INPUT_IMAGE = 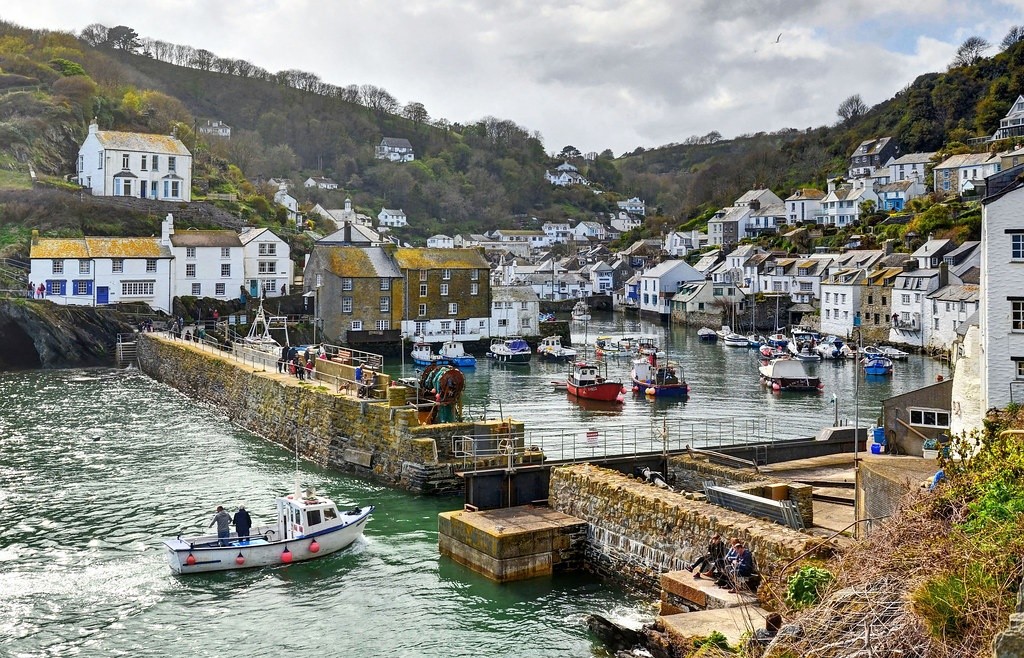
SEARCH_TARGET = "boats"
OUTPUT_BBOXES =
[439,330,478,368]
[565,360,623,401]
[245,290,306,359]
[758,351,821,393]
[716,326,731,339]
[548,380,568,391]
[630,352,688,398]
[489,335,532,366]
[787,323,910,360]
[571,302,592,321]
[698,325,718,343]
[410,336,449,368]
[161,432,375,576]
[862,356,895,375]
[596,336,665,359]
[537,334,578,362]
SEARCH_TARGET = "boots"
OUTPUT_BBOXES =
[703,570,713,577]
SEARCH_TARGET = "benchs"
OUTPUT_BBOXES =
[364,357,381,370]
[331,350,351,364]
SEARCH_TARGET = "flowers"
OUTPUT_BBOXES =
[922,437,942,451]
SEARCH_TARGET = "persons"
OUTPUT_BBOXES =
[262,288,267,299]
[807,337,816,356]
[232,505,251,543]
[277,342,326,380]
[185,330,192,341]
[281,284,287,296]
[797,340,803,353]
[685,534,754,594]
[167,316,184,338]
[199,327,206,345]
[138,317,155,332]
[355,363,379,398]
[29,281,45,299]
[209,506,232,547]
[213,309,219,329]
[193,325,201,343]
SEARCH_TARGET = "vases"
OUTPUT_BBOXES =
[923,449,939,460]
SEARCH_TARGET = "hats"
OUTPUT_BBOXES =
[238,503,245,509]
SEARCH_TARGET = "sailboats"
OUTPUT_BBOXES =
[396,335,421,388]
[725,281,791,349]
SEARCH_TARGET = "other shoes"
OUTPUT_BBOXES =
[714,579,727,586]
[693,572,700,578]
[685,567,694,573]
[728,588,736,593]
[722,585,729,589]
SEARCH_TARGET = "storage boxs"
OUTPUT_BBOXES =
[873,426,885,443]
[871,444,884,454]
